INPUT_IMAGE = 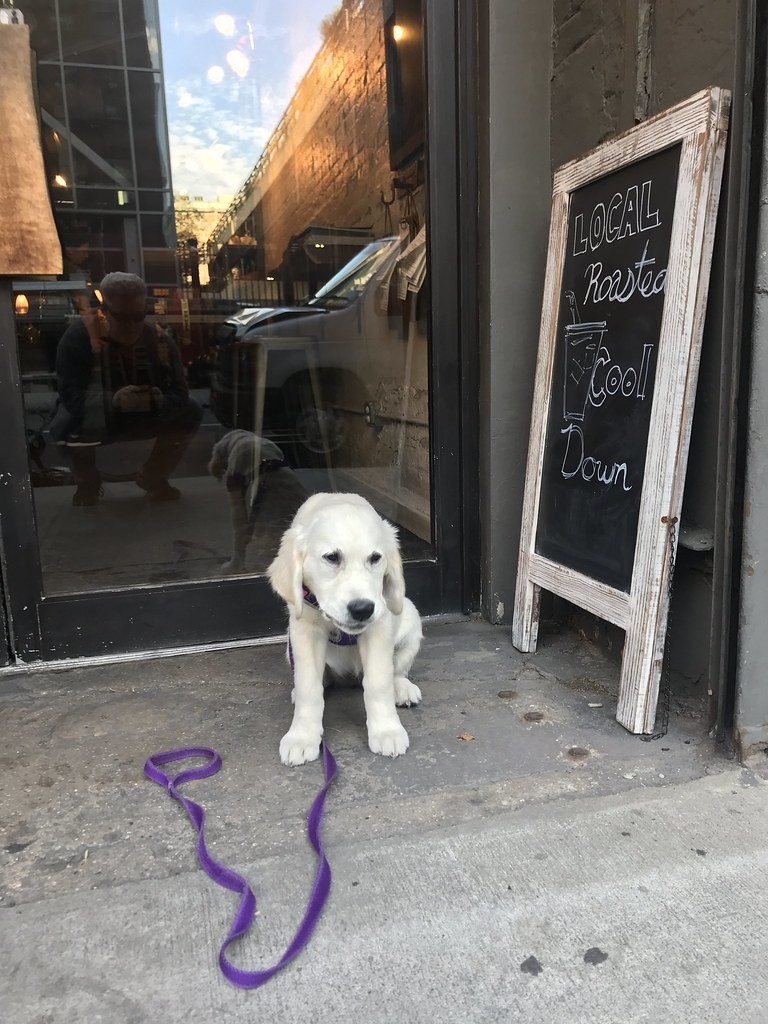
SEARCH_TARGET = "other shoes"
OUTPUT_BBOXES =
[135,464,182,500]
[72,474,105,507]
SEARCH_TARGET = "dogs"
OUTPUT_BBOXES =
[266,492,425,770]
[209,428,307,576]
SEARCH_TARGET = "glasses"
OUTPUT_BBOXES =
[103,302,149,323]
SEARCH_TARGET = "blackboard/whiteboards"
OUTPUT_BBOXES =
[508,82,735,633]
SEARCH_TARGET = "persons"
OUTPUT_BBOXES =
[51,272,204,505]
